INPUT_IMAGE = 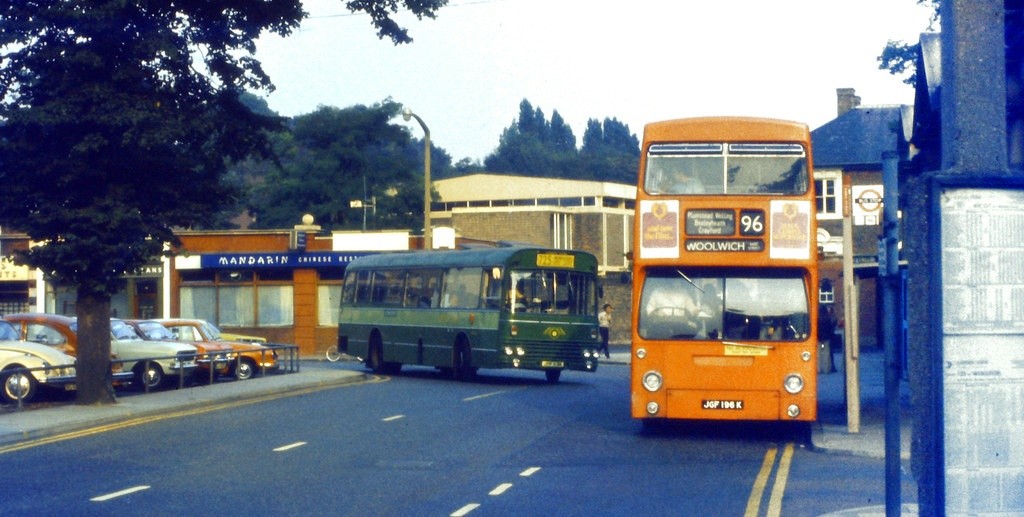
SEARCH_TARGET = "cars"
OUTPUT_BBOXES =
[69,317,199,389]
[125,318,279,380]
[0,319,78,403]
[3,314,124,390]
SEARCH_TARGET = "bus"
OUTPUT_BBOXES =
[336,239,603,381]
[626,116,831,444]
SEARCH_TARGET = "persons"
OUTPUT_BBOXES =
[597,304,613,358]
[492,280,524,306]
[701,284,721,312]
[817,305,837,374]
[667,164,705,194]
[718,282,761,340]
[646,278,698,319]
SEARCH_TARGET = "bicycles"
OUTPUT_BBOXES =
[326,336,363,363]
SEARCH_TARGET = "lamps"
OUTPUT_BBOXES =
[301,213,315,225]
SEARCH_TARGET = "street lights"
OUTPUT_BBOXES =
[402,107,431,250]
